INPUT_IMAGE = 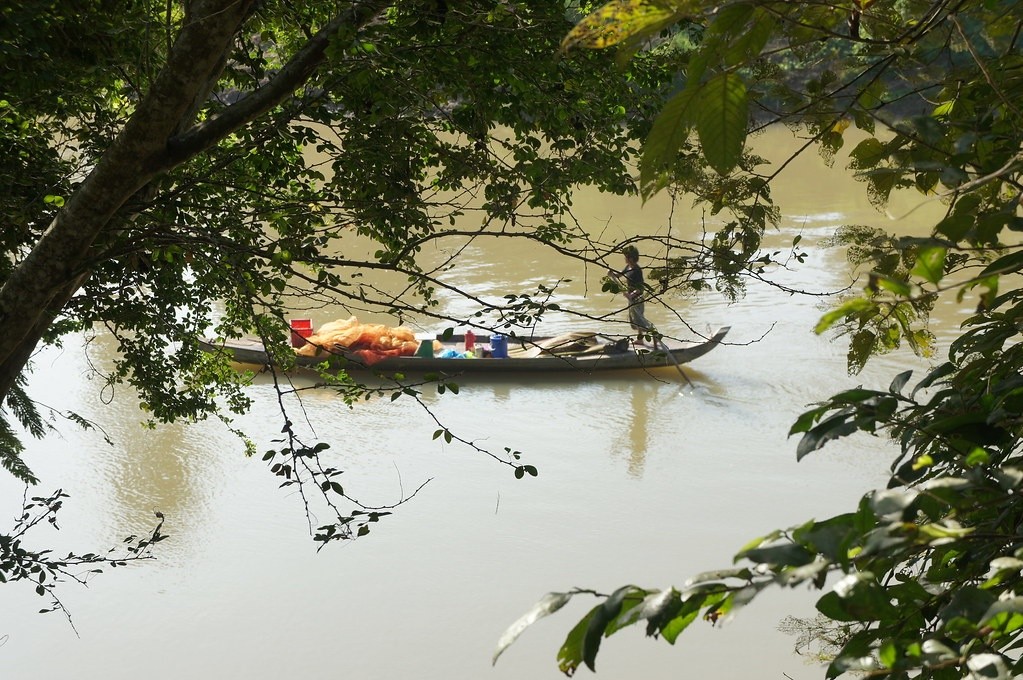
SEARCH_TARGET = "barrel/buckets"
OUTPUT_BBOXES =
[490,334,508,358]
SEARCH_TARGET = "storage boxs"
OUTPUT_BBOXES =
[289,318,313,348]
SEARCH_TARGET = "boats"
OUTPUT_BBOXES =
[195,325,731,374]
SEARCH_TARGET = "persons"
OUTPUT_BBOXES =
[607,247,658,349]
